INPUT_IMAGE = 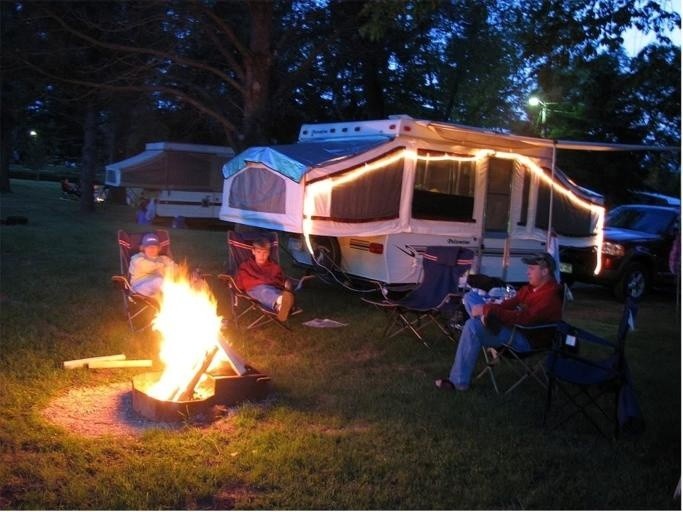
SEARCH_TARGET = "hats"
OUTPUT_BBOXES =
[521,252,555,270]
[142,233,160,245]
[254,237,272,248]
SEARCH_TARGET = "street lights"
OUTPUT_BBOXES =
[527,96,546,137]
[28,129,40,182]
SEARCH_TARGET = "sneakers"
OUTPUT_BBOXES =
[276,291,303,322]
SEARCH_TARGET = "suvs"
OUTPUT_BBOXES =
[559,204,681,302]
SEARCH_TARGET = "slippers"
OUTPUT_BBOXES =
[434,379,454,390]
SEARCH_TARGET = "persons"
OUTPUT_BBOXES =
[127,233,181,299]
[62,178,81,199]
[235,236,296,322]
[432,251,563,392]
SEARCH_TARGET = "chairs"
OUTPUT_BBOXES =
[378,246,474,351]
[472,284,567,398]
[111,230,173,333]
[544,295,642,443]
[218,229,316,335]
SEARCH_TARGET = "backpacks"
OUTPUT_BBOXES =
[1,216,28,225]
